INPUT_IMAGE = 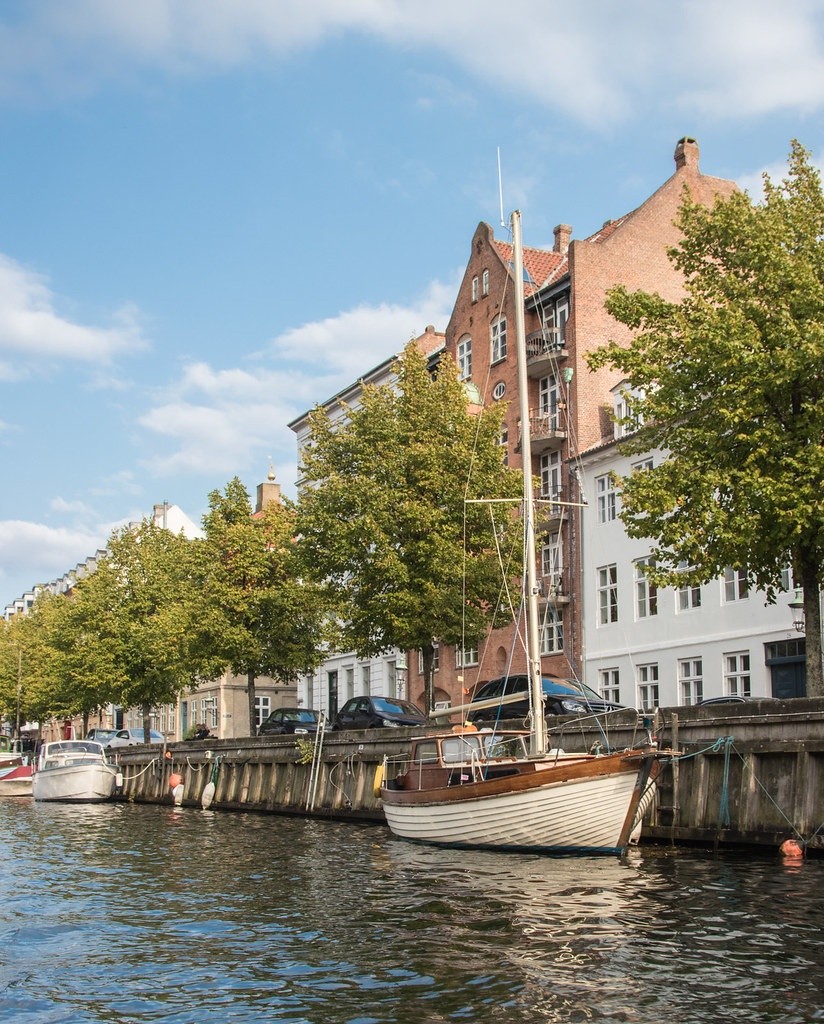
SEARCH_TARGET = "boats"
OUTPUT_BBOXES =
[1,754,33,796]
[31,739,119,802]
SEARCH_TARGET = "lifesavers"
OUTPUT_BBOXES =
[373,764,384,798]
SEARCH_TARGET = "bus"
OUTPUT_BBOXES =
[0,734,11,754]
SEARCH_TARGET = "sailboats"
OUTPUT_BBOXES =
[374,200,688,852]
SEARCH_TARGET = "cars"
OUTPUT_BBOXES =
[106,727,173,748]
[84,728,121,747]
[256,708,332,736]
[694,696,781,706]
[332,696,431,732]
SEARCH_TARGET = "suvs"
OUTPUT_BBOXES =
[465,673,629,723]
